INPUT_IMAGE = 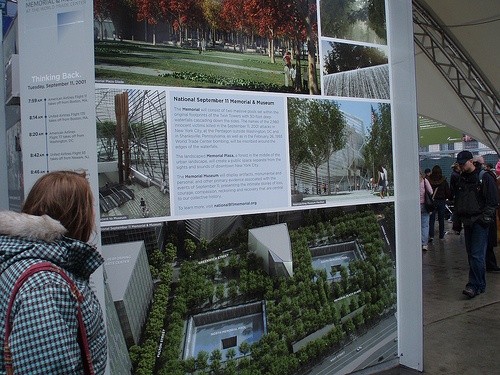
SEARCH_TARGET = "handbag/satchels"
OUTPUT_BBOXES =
[425,190,435,212]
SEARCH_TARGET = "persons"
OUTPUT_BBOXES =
[289,64,296,89]
[419,167,433,250]
[114,139,172,217]
[376,166,385,199]
[452,150,499,298]
[423,168,431,181]
[0,171,108,375]
[94,19,282,59]
[428,165,452,242]
[301,67,309,91]
[293,165,373,199]
[283,51,291,87]
[473,154,500,271]
[449,163,462,208]
[381,166,389,198]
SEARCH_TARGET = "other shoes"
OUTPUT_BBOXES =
[439,237,446,240]
[422,245,428,250]
[455,231,460,235]
[462,282,486,297]
[429,238,433,242]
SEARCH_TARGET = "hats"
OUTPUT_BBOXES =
[456,150,473,164]
